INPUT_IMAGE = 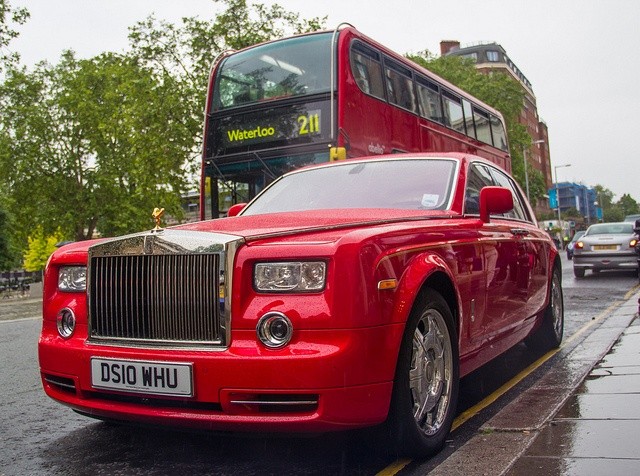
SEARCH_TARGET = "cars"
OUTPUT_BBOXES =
[573,221,640,278]
[37,151,565,459]
[566,231,586,260]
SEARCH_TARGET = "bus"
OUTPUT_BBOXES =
[198,22,513,221]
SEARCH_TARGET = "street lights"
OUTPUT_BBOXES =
[553,163,573,225]
[521,139,545,202]
